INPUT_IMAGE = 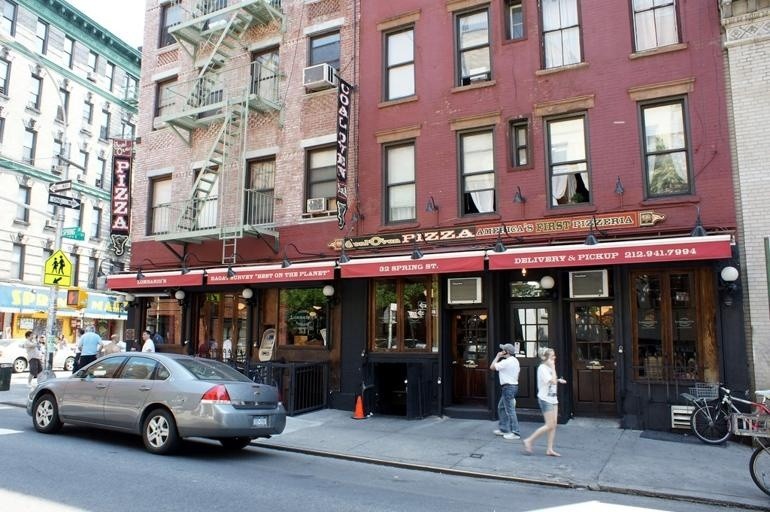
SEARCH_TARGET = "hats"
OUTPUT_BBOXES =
[499,343,514,354]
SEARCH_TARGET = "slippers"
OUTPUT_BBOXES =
[546,452,561,457]
[522,439,535,455]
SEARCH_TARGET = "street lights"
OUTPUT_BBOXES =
[1,29,72,385]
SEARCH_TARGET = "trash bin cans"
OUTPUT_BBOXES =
[0,363,12,390]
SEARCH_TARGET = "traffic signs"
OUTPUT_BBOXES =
[417,310,425,317]
[47,179,73,194]
[65,232,85,240]
[418,302,427,309]
[60,227,82,236]
[47,195,82,211]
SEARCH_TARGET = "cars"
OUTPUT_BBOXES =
[0,333,75,373]
[375,338,427,350]
[20,350,289,456]
[70,338,126,371]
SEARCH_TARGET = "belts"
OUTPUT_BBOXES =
[501,384,517,387]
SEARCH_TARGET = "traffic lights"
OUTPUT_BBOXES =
[66,286,89,310]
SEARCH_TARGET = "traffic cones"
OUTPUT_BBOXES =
[349,393,367,420]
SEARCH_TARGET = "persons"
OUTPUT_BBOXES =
[523,347,567,456]
[21,322,163,379]
[200,335,233,361]
[490,344,522,440]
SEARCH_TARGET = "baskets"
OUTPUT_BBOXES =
[680,382,720,401]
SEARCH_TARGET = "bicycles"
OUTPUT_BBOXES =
[680,377,770,445]
[742,427,770,497]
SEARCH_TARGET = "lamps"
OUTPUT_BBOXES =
[322,284,337,307]
[118,293,138,311]
[494,222,523,253]
[425,174,624,215]
[175,290,189,307]
[687,204,707,238]
[96,237,382,282]
[583,216,608,246]
[411,231,429,259]
[719,262,743,307]
[540,275,555,296]
[242,289,255,305]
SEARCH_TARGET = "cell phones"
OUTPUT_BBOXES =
[503,351,507,355]
[547,359,550,362]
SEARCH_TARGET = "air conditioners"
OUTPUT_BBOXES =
[302,62,335,90]
[307,198,328,212]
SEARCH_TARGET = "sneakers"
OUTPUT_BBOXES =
[493,429,508,435]
[503,433,520,440]
[27,384,32,388]
